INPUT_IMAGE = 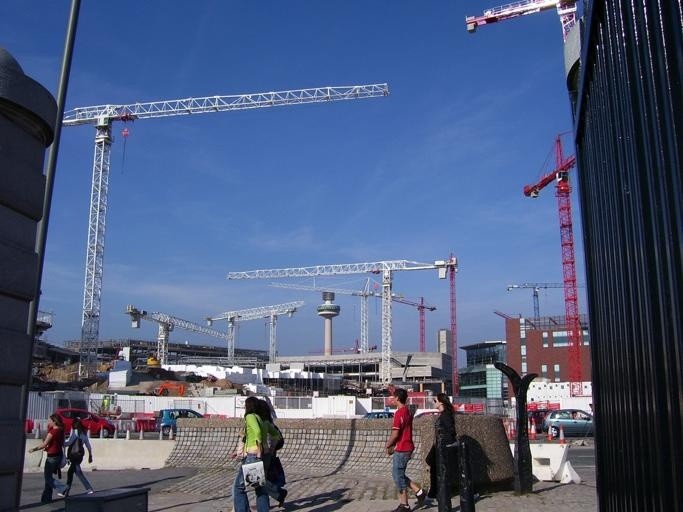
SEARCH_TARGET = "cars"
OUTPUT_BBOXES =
[530,409,594,437]
[155,409,206,436]
[362,412,394,419]
[47,407,115,438]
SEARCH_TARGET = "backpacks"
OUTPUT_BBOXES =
[252,412,285,458]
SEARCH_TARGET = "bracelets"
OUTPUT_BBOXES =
[32,447,40,451]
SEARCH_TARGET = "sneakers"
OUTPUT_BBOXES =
[391,503,411,512]
[415,487,427,507]
[57,485,95,497]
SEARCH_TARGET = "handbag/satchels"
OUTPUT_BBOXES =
[241,460,267,492]
[66,435,85,463]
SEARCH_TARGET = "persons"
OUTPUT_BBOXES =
[239,400,288,510]
[385,387,427,512]
[232,396,269,512]
[431,393,476,512]
[57,419,94,498]
[28,414,69,504]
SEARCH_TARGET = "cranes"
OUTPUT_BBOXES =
[124,305,227,367]
[225,258,437,392]
[464,0,582,41]
[268,277,377,354]
[493,130,583,404]
[205,301,306,363]
[434,251,461,397]
[61,79,392,391]
[393,294,437,352]
